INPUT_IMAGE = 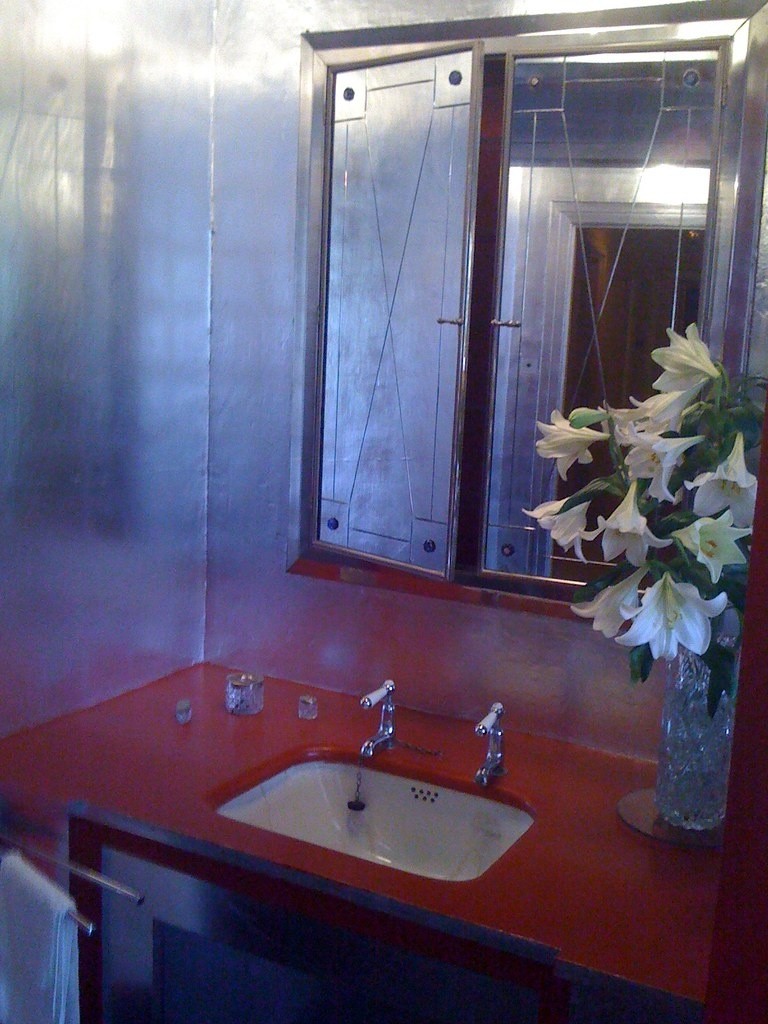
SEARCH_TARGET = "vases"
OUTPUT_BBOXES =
[654,599,744,829]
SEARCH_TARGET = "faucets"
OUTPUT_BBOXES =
[359,677,396,756]
[473,701,509,786]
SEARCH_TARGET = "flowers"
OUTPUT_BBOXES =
[523,316,767,715]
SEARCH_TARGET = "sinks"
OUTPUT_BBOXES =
[216,743,535,882]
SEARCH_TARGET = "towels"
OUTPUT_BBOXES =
[1,854,80,1024]
